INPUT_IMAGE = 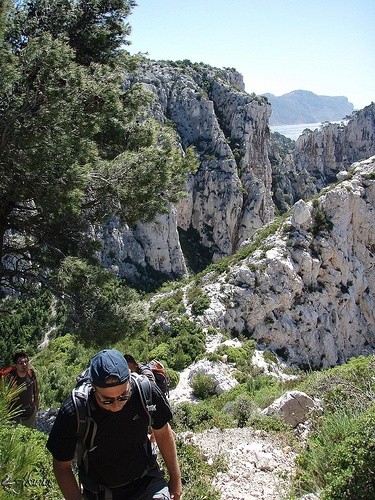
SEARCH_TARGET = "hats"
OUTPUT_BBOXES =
[88,350,130,388]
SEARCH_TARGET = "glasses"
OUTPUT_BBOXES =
[17,360,28,365]
[95,389,130,405]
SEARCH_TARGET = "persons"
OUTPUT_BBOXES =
[123,354,157,450]
[46,349,182,500]
[4,352,40,428]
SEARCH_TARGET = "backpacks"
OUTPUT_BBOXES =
[72,367,161,497]
[138,359,167,393]
[0,365,34,394]
